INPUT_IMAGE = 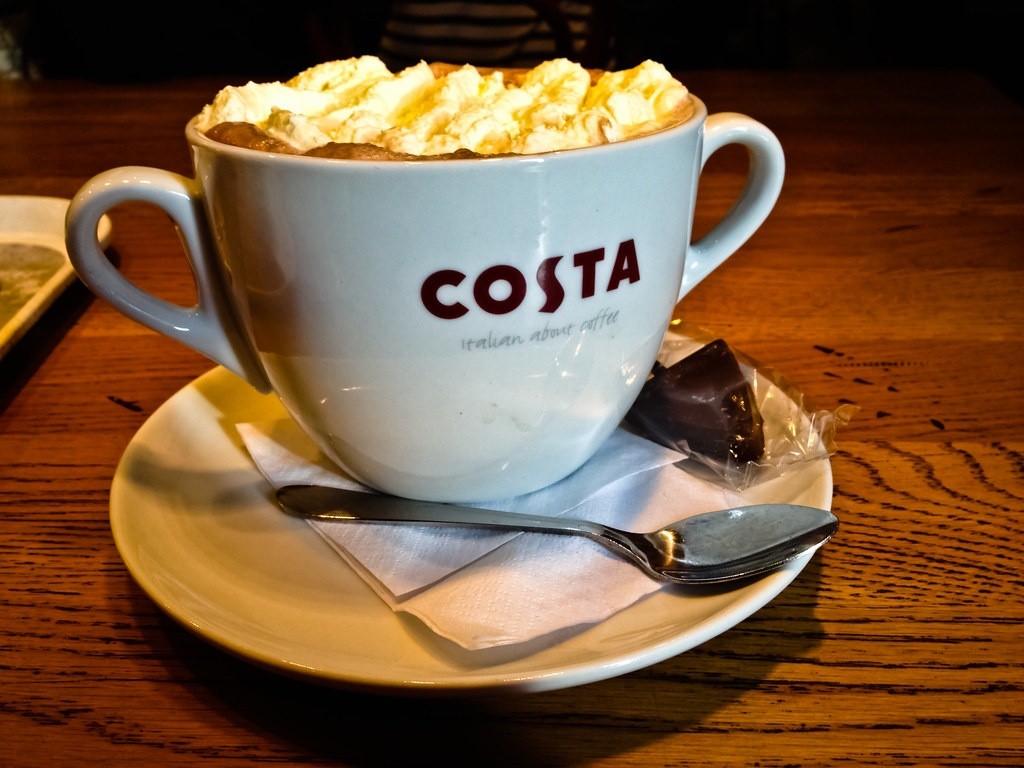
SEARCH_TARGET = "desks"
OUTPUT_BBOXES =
[1,76,1024,768]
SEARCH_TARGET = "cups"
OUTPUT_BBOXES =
[65,91,786,503]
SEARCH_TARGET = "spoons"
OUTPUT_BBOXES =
[275,483,842,587]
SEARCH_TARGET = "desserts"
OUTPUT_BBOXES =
[619,338,767,465]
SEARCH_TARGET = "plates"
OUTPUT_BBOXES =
[0,195,112,355]
[107,330,835,699]
